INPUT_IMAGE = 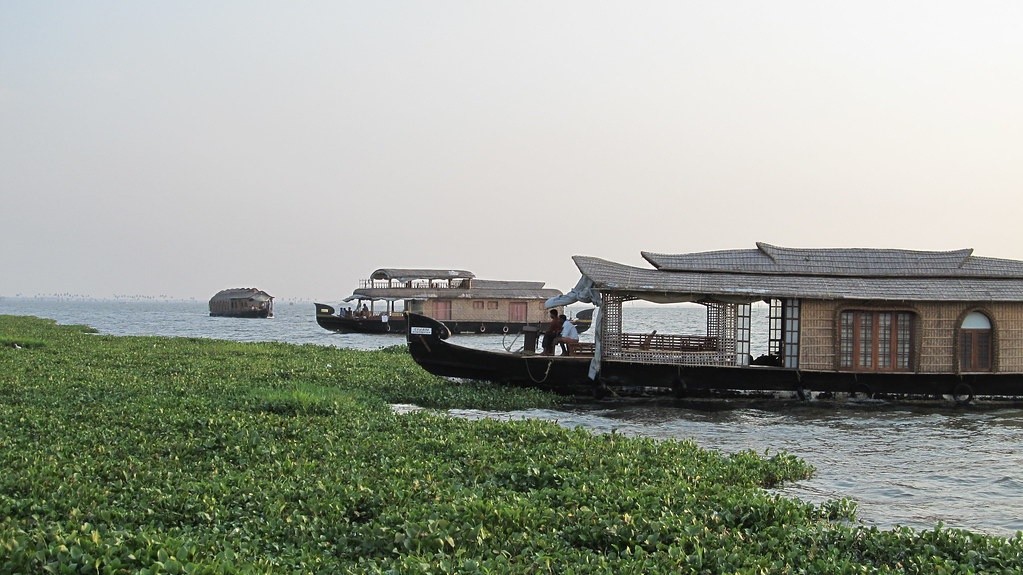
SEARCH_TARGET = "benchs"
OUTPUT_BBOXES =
[567,329,718,357]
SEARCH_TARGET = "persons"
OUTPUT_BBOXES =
[544,313,579,356]
[540,308,563,356]
[340,303,370,319]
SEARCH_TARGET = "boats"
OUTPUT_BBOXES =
[314,268,597,338]
[208,287,274,318]
[400,239,1023,408]
